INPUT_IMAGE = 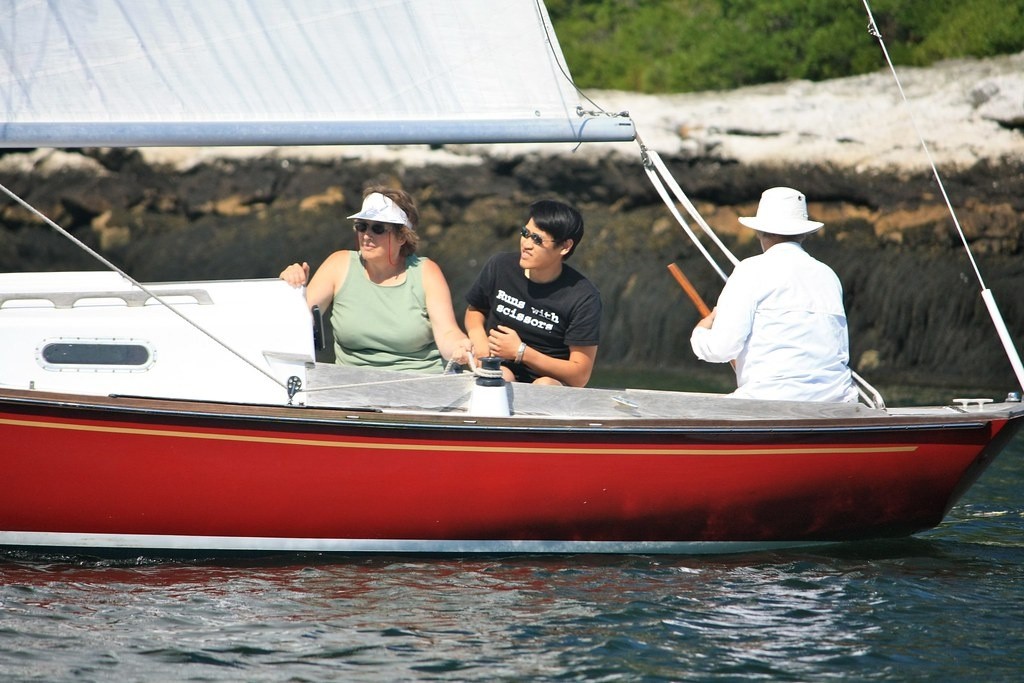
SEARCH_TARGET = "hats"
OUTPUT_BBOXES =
[738,186,824,235]
[347,192,413,231]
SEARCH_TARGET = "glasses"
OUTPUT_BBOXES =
[520,226,564,246]
[354,222,402,235]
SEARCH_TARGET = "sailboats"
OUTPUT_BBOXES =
[0,1,1023,558]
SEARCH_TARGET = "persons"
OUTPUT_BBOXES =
[464,200,602,388]
[690,186,858,402]
[279,186,475,375]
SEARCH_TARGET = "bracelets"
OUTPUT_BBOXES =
[514,342,526,364]
